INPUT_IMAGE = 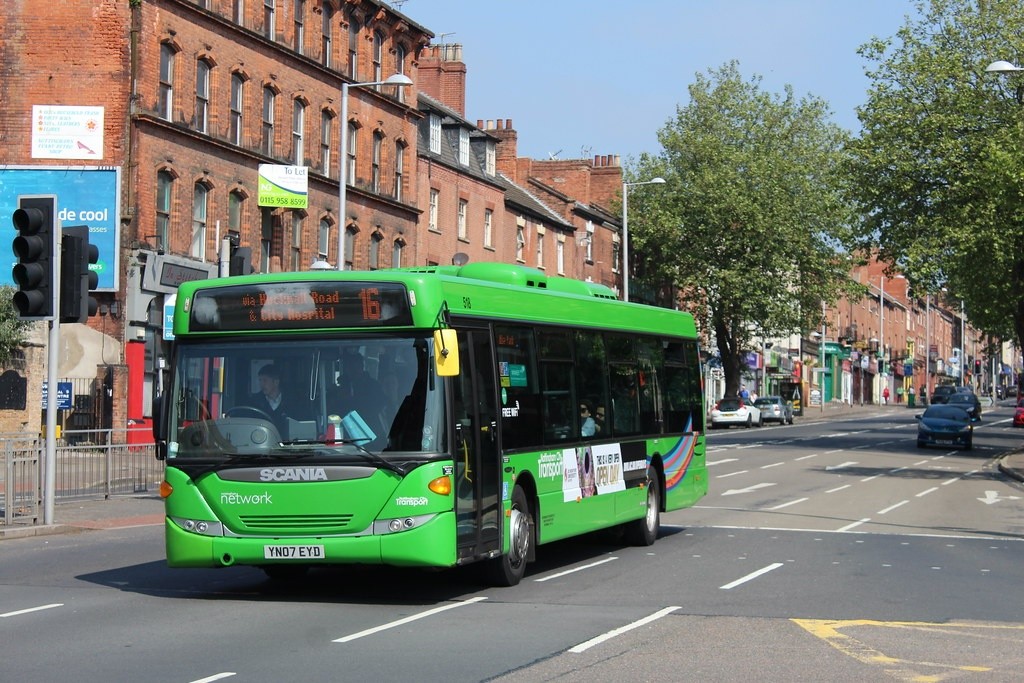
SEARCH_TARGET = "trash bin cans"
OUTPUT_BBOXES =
[908,394,914,406]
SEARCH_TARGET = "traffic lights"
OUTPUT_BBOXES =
[974,359,981,376]
[61,223,98,322]
[12,194,53,321]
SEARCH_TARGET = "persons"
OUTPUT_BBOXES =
[738,384,757,403]
[223,363,309,443]
[897,384,926,403]
[332,352,647,448]
[883,386,889,405]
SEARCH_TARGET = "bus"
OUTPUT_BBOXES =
[157,260,708,587]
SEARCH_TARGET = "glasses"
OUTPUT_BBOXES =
[581,408,588,413]
[595,412,605,418]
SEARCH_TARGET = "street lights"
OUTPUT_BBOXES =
[619,175,668,302]
[339,73,415,267]
[878,273,908,406]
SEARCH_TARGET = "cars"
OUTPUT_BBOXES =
[752,396,794,426]
[712,397,763,429]
[929,385,981,421]
[914,403,974,449]
